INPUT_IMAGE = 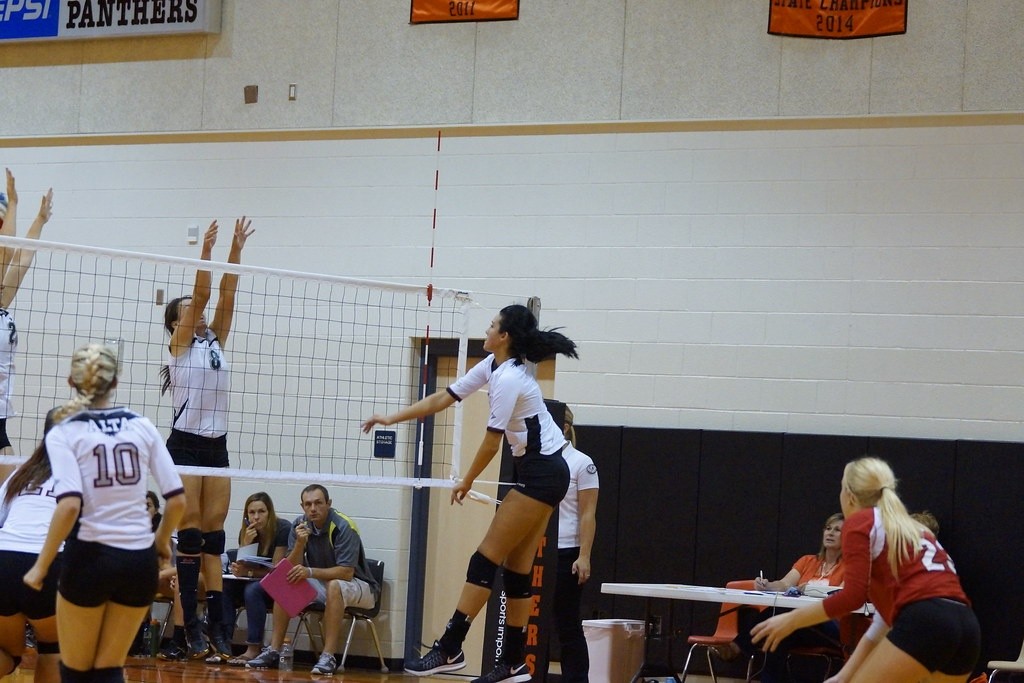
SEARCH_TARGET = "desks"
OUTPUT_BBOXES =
[600,582,876,683]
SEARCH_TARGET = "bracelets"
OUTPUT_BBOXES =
[307,567,312,578]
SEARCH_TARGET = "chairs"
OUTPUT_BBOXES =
[133,536,389,675]
[680,577,1024,683]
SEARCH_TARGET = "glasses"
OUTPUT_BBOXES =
[210,350,220,370]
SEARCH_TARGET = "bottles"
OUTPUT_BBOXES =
[279,638,293,672]
[146,619,160,656]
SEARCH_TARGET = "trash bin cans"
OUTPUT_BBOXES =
[581,619,645,683]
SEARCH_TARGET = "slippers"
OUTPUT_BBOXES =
[204,655,224,664]
[228,655,249,667]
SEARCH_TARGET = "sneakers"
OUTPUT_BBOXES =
[310,652,336,675]
[184,617,208,658]
[157,647,186,660]
[470,656,532,683]
[208,622,231,659]
[404,640,467,676]
[245,646,279,669]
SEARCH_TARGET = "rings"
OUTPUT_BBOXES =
[587,575,590,577]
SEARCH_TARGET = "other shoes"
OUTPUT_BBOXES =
[710,642,739,664]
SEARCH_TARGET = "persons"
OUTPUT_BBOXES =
[159,216,256,658]
[362,304,578,683]
[707,513,846,663]
[543,404,599,683]
[750,456,982,683]
[0,404,64,683]
[0,165,53,487]
[246,485,381,677]
[910,511,939,535]
[126,491,207,663]
[23,343,186,683]
[206,492,292,666]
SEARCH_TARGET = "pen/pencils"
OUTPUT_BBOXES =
[743,592,763,596]
[760,570,762,581]
[244,518,249,526]
